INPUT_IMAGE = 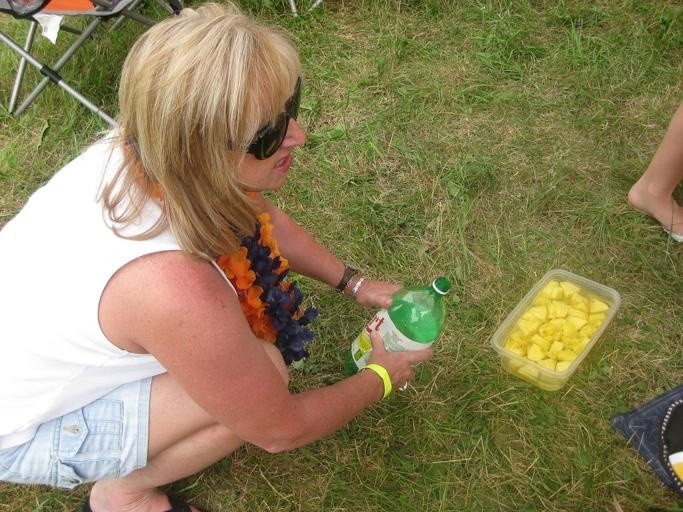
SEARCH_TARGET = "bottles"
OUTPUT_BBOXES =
[343,277,451,376]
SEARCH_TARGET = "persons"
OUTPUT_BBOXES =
[626,97,682,245]
[0,0,438,511]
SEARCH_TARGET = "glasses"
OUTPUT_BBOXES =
[227,74,302,161]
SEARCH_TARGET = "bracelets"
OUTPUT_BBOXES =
[347,275,369,300]
[333,257,361,291]
[360,362,394,402]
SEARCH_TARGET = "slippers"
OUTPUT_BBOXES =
[83,493,211,512]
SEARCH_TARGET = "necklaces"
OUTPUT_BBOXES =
[121,126,320,367]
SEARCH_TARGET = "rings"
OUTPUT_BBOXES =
[397,381,408,391]
